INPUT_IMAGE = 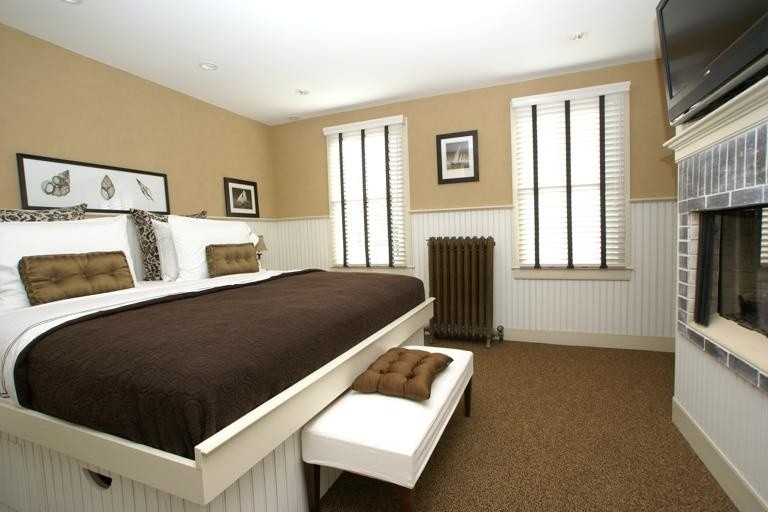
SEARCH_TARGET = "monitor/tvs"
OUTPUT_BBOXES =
[655,1,768,126]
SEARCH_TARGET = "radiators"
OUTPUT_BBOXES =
[424,236,504,348]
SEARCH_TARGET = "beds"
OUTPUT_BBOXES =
[0,269,437,512]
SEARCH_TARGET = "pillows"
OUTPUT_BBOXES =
[0,203,260,308]
[351,346,454,400]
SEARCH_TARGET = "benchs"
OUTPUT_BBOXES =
[299,344,473,512]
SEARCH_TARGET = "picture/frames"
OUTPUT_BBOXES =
[223,177,259,218]
[16,153,170,215]
[436,130,479,184]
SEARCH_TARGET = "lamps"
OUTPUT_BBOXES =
[255,236,268,260]
